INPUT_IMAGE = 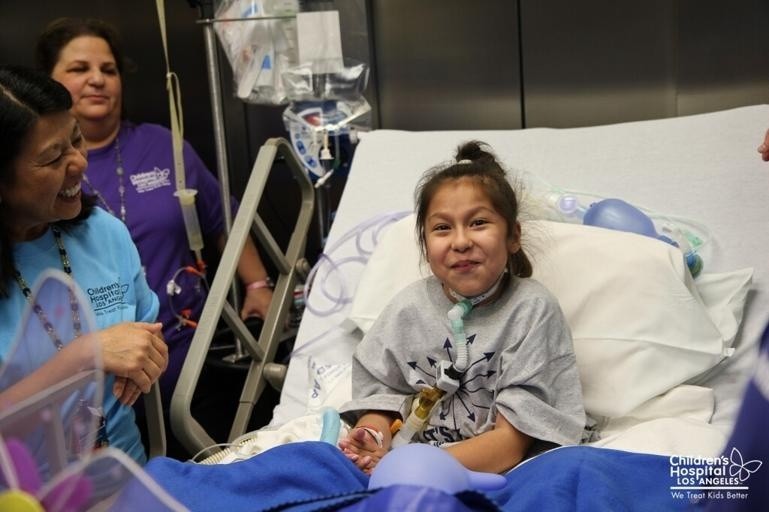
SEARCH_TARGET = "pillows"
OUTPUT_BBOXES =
[353,211,735,420]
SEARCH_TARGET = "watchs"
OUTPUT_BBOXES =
[247,276,275,290]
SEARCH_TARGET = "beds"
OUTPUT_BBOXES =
[0,104,769,511]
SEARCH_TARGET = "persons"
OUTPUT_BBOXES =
[339,140,586,474]
[34,16,273,393]
[0,65,169,512]
